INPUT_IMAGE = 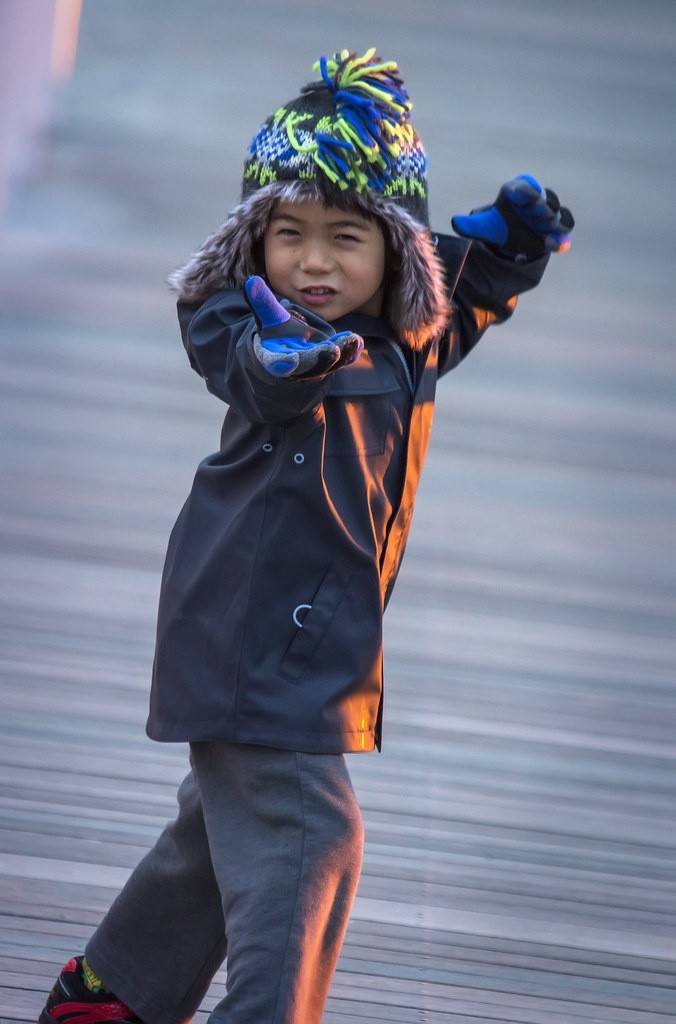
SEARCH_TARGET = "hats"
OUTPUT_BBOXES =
[167,46,451,353]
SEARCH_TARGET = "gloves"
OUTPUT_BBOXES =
[450,174,575,262]
[245,277,364,377]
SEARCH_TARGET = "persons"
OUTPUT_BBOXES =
[36,46,577,1024]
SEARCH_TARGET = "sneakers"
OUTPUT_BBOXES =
[40,957,142,1024]
